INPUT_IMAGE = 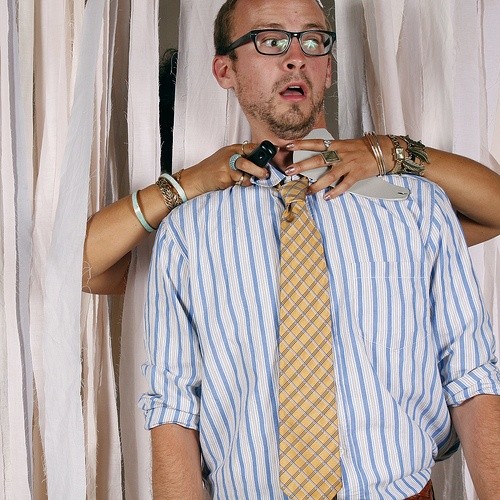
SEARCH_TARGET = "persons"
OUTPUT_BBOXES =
[82,48,500,297]
[144,0,500,500]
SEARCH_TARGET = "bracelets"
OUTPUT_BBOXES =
[398,135,431,178]
[132,188,158,234]
[155,167,188,211]
[363,131,386,177]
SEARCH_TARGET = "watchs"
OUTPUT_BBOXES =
[386,134,405,175]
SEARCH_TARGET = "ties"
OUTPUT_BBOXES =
[277,176,342,500]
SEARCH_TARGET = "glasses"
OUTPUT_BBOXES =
[228,28,336,57]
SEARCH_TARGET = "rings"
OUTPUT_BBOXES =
[235,173,245,185]
[320,150,342,164]
[229,153,243,168]
[322,138,333,150]
[241,138,248,153]
